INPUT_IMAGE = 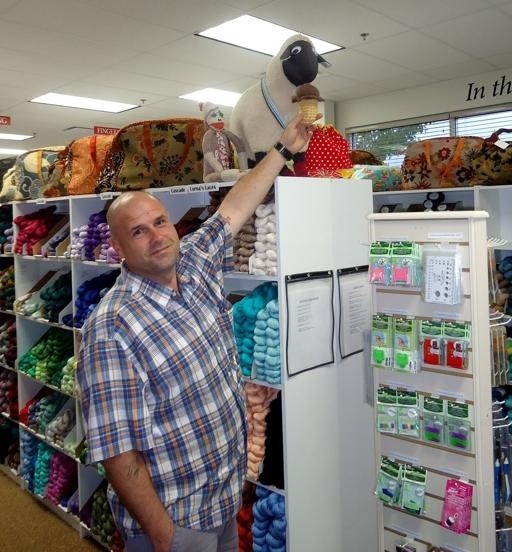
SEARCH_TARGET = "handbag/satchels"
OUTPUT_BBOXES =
[95,115,216,193]
[400,135,489,190]
[0,132,114,203]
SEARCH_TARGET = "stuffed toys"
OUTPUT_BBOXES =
[199,108,253,185]
[225,33,333,172]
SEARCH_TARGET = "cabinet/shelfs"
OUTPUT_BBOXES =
[0,174,371,551]
[371,187,512,551]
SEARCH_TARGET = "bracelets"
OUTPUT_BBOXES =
[273,142,293,161]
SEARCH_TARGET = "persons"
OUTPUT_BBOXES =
[70,110,328,552]
